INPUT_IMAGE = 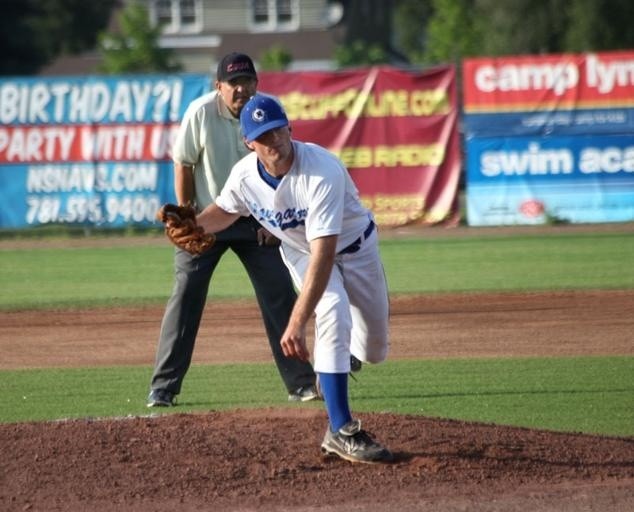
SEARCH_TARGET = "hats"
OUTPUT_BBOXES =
[215,51,257,83]
[237,94,289,143]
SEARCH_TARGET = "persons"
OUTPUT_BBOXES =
[165,97,392,465]
[146,51,324,407]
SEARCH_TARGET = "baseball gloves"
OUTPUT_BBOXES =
[158,203,218,256]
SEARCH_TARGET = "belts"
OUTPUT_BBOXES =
[335,220,375,255]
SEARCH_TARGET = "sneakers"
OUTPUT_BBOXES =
[146,388,176,407]
[349,353,361,371]
[286,383,318,402]
[319,421,394,465]
